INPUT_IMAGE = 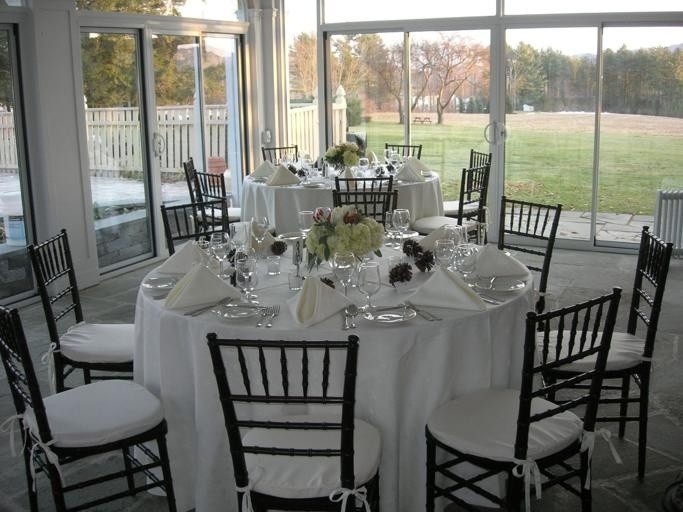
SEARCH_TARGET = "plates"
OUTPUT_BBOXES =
[386,231,419,239]
[362,309,416,324]
[252,177,266,182]
[421,171,431,177]
[475,277,526,291]
[279,231,302,241]
[215,303,258,320]
[142,278,178,290]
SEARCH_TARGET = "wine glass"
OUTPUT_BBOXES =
[332,251,355,297]
[196,222,259,305]
[250,217,268,260]
[386,209,410,250]
[357,262,381,310]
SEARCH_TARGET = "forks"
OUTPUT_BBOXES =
[405,300,443,321]
[255,304,280,327]
[471,286,505,302]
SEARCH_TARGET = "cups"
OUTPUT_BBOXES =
[435,224,478,288]
[287,272,302,295]
[266,255,281,275]
[298,211,313,240]
[271,148,403,188]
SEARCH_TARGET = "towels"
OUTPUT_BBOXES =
[401,268,488,316]
[286,275,354,329]
[162,264,248,311]
[156,238,223,273]
[475,247,534,279]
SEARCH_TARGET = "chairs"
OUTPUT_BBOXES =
[24,227,147,393]
[496,194,564,333]
[530,224,675,485]
[425,285,622,512]
[204,332,384,511]
[0,305,177,511]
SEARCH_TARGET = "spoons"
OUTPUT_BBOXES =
[183,296,231,316]
[348,303,358,328]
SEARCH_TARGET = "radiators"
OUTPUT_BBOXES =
[652,190,683,258]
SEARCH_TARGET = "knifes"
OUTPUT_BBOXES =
[342,305,350,329]
